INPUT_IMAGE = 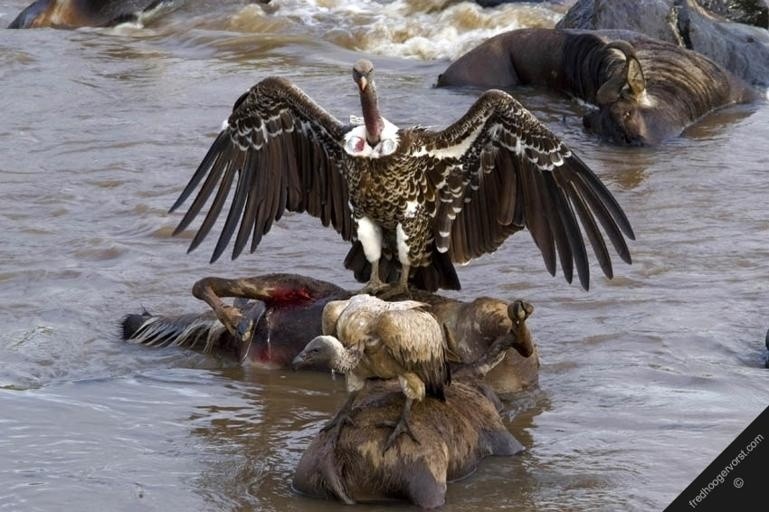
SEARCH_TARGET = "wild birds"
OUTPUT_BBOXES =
[167,59,636,299]
[293,294,451,456]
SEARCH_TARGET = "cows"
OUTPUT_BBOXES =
[293,347,540,508]
[122,272,540,395]
[434,29,769,147]
